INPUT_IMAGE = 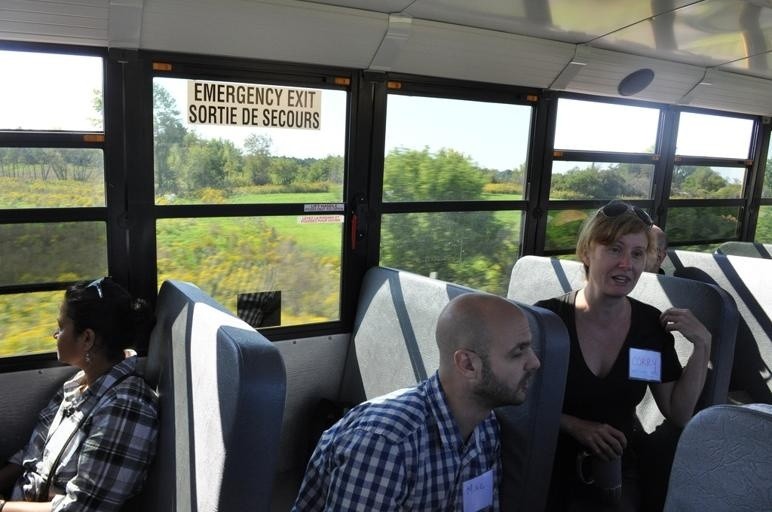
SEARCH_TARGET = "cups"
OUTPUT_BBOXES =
[575,449,623,496]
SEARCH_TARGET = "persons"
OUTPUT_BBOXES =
[0,274,161,512]
[530,198,714,511]
[645,223,669,277]
[289,291,543,512]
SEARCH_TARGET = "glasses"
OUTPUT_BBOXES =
[603,202,655,228]
[86,276,113,299]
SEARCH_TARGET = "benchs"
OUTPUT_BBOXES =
[505,255,739,416]
[720,240,772,259]
[665,404,771,511]
[660,250,772,403]
[144,281,286,512]
[335,267,571,512]
